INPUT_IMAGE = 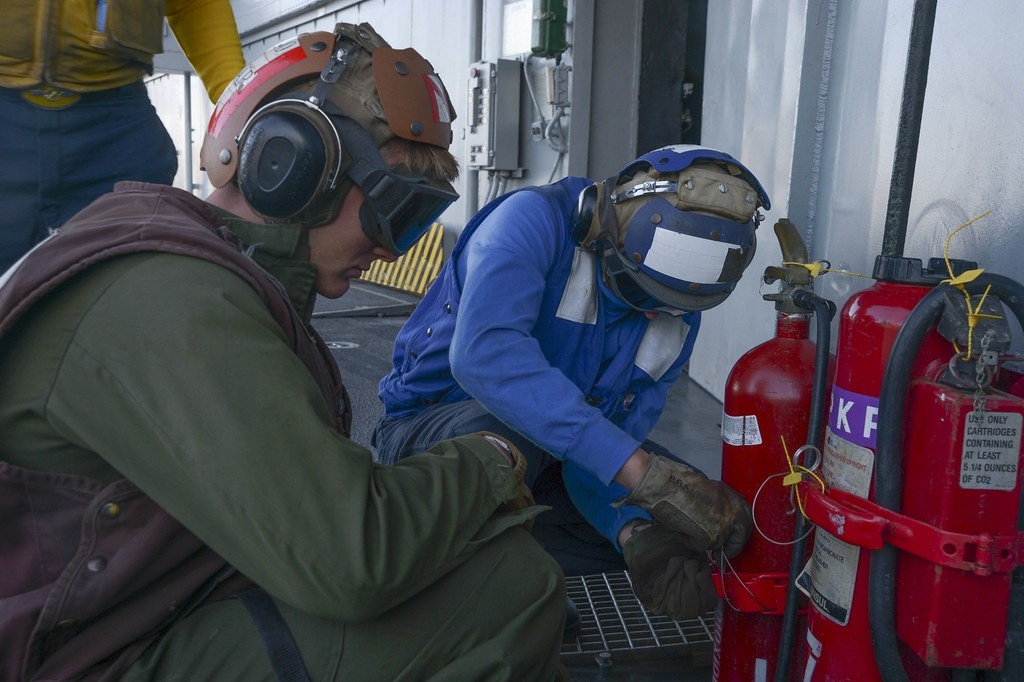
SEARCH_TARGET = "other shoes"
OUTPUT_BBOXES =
[563,594,582,640]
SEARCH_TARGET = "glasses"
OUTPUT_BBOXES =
[607,270,737,316]
[362,168,460,256]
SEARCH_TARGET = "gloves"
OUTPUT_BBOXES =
[622,519,720,621]
[475,431,536,532]
[611,451,752,564]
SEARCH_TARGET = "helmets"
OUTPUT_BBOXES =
[569,143,771,317]
[201,23,457,228]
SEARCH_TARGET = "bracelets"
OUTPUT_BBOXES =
[483,435,516,468]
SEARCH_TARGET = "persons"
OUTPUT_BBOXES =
[0,0,244,279]
[375,144,770,641]
[0,23,567,682]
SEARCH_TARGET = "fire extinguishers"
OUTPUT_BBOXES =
[717,218,1024,682]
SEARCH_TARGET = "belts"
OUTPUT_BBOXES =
[0,81,148,110]
